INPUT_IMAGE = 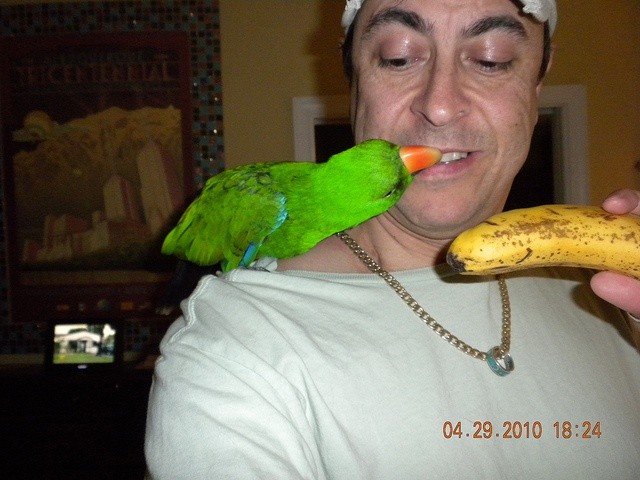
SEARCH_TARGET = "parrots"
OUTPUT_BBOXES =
[160,139,443,274]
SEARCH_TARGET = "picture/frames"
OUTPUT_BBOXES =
[0,30,196,324]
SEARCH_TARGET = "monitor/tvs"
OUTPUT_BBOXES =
[44,317,123,370]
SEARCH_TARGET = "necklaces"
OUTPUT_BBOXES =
[335,230,514,376]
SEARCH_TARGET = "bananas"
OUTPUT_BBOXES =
[445,205,640,279]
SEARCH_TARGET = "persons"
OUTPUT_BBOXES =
[142,0,640,480]
